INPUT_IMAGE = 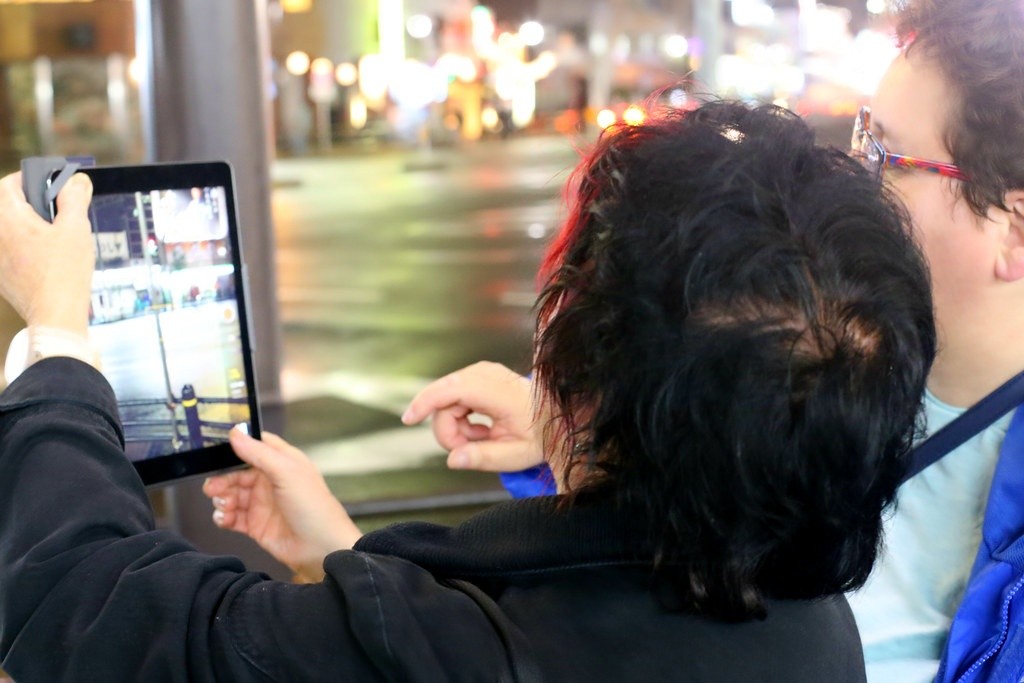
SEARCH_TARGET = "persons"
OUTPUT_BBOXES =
[0,100,935,683]
[185,185,215,236]
[400,0,1024,683]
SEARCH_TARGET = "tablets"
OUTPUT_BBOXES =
[45,159,263,488]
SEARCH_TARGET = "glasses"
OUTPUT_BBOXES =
[851,106,977,191]
